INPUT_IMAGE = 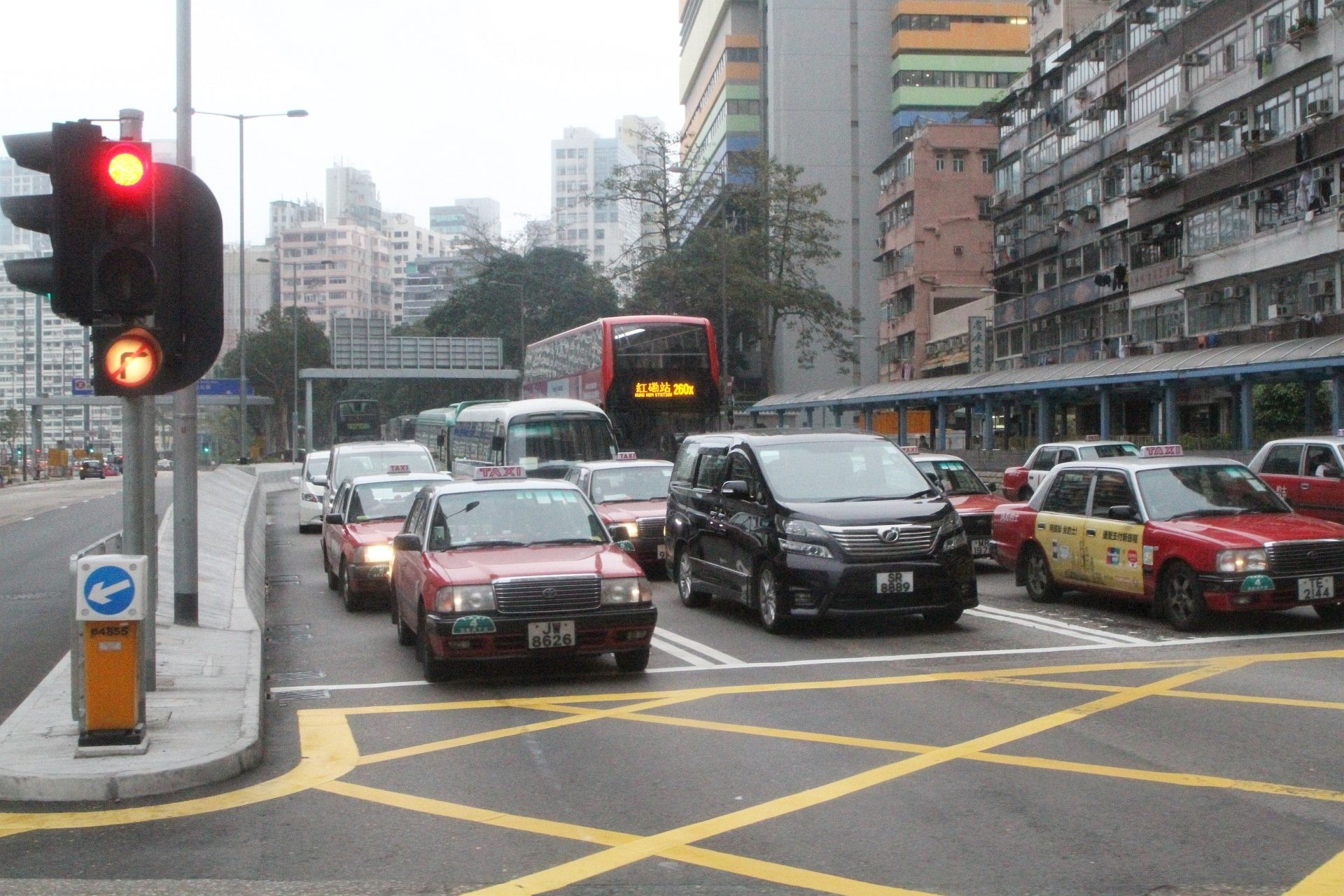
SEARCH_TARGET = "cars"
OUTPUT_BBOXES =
[664,427,980,639]
[290,449,379,534]
[1002,439,1144,499]
[393,465,659,681]
[893,444,1011,560]
[79,461,105,480]
[989,440,1344,630]
[1244,436,1344,519]
[323,471,460,613]
[564,451,677,578]
[323,440,440,518]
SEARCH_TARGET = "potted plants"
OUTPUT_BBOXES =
[1288,14,1316,39]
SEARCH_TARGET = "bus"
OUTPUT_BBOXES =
[523,315,725,461]
[106,452,122,472]
[330,397,379,446]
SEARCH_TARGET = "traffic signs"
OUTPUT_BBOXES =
[74,555,148,620]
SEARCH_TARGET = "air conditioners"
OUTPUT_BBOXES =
[1177,257,1190,270]
[1189,124,1209,141]
[1312,166,1333,182]
[1224,286,1237,299]
[904,335,969,356]
[954,246,963,254]
[988,9,1147,371]
[1330,193,1344,206]
[1198,292,1214,304]
[1308,279,1332,297]
[1324,0,1343,9]
[1151,223,1165,238]
[876,237,901,257]
[1248,189,1270,202]
[1158,91,1189,127]
[1229,110,1242,124]
[1233,195,1248,208]
[1240,128,1264,145]
[1177,53,1199,66]
[1307,99,1333,118]
[1163,140,1180,154]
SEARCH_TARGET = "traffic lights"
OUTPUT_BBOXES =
[18,448,21,456]
[89,142,160,314]
[36,448,40,453]
[1,118,87,322]
[176,101,309,462]
[203,442,208,453]
[88,444,94,453]
[92,324,166,395]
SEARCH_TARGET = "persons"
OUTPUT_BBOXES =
[919,435,929,448]
[1315,448,1339,477]
[358,486,386,516]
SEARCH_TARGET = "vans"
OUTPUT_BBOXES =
[381,407,452,470]
[452,396,620,482]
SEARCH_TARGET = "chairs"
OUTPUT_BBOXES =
[685,346,702,368]
[622,346,638,369]
[350,487,384,522]
[339,458,365,485]
[311,463,325,475]
[662,349,683,369]
[815,455,854,497]
[1267,457,1292,474]
[1307,453,1330,479]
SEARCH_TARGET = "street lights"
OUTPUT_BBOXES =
[257,257,335,460]
